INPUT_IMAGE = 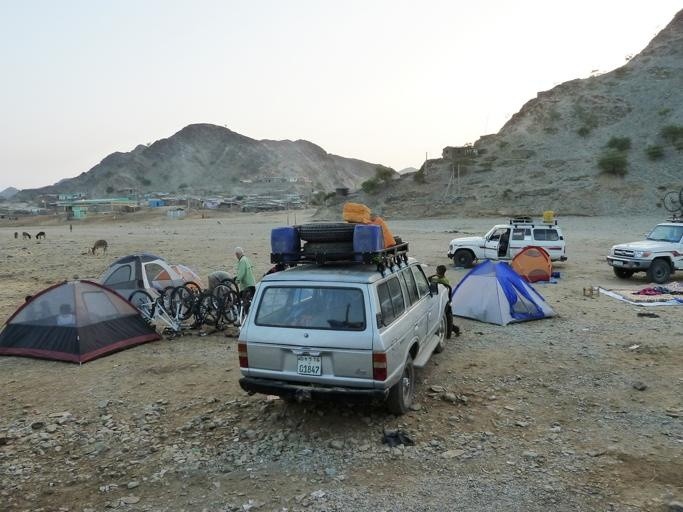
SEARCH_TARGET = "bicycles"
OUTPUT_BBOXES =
[658,187,682,217]
[124,277,259,341]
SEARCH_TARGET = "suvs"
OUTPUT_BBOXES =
[604,216,682,284]
[439,214,570,268]
[233,211,452,419]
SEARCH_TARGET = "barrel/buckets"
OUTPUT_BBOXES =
[543,211,554,223]
[353,225,384,253]
[271,227,301,261]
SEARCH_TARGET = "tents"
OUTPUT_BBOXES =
[0,279,164,366]
[93,252,185,315]
[449,258,560,327]
[508,245,553,284]
[152,265,204,291]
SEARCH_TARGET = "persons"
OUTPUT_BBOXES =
[427,265,460,339]
[57,303,74,327]
[233,247,255,311]
[207,270,237,310]
[39,299,51,319]
[24,295,35,321]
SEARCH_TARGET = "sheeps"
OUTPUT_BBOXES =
[35,232,46,239]
[91,239,109,256]
[22,232,31,240]
[14,232,18,239]
